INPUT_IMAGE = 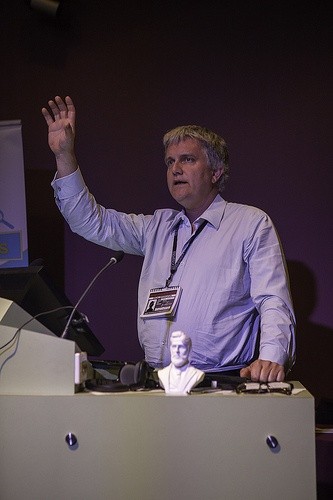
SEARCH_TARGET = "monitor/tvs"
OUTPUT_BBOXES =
[0,264,106,357]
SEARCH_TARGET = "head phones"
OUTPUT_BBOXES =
[85,361,151,392]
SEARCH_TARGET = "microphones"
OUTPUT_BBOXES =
[60,250,125,339]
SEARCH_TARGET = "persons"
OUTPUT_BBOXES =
[158,331,205,397]
[42,96,297,384]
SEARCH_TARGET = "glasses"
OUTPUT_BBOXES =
[235,381,293,396]
